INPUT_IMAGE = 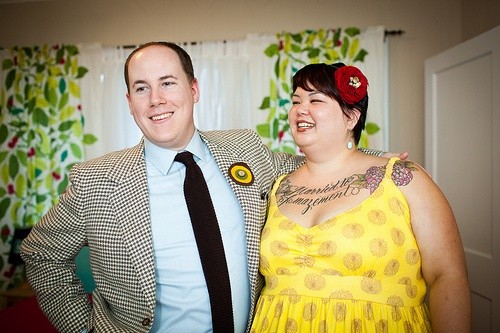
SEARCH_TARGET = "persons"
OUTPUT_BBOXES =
[248,63,473,333]
[19,42,408,333]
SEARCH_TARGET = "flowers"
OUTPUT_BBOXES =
[333,66,369,105]
